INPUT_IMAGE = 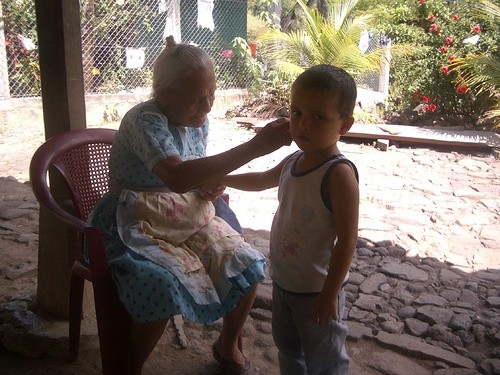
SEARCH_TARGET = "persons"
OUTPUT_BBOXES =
[86,44,292,375]
[204,65,360,375]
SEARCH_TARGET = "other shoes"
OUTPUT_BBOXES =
[212,340,260,375]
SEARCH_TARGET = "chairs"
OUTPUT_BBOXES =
[30,128,247,375]
